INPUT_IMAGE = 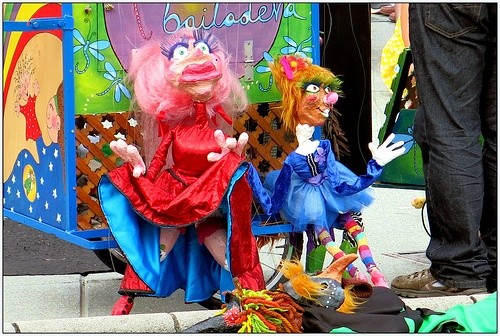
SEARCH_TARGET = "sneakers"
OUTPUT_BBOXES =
[391,269,488,298]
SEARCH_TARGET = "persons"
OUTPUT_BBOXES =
[390,2,498,297]
[265,57,406,288]
[97,25,267,315]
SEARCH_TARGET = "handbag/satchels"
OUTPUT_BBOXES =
[294,282,423,334]
